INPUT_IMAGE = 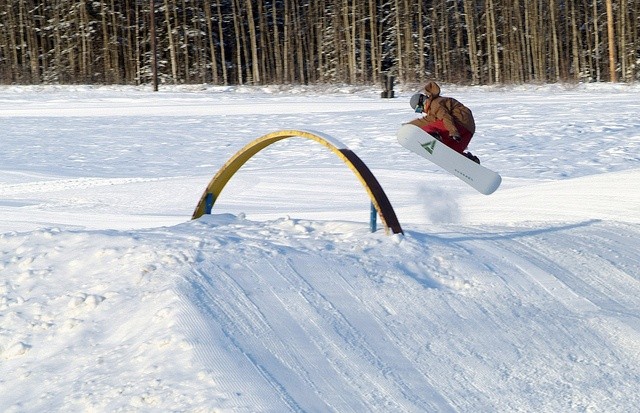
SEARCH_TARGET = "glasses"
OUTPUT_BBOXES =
[415,94,424,113]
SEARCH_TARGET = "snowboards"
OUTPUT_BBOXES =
[397,123,502,195]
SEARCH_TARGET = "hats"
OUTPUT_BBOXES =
[410,93,428,110]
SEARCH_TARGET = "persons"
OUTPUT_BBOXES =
[405,82,480,164]
